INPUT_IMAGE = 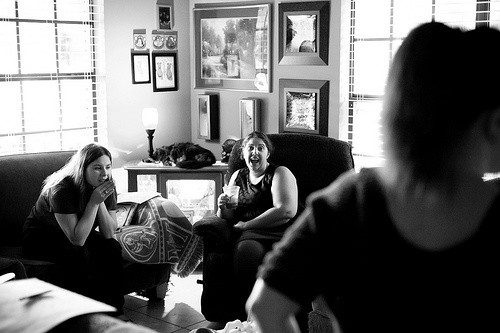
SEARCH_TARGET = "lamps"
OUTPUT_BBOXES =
[141,108,159,159]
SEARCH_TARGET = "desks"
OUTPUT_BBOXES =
[123,157,229,226]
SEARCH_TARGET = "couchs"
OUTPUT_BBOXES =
[0,149,201,311]
[192,132,355,325]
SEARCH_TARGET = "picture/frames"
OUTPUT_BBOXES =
[197,93,221,141]
[278,1,331,68]
[131,50,152,84]
[278,78,330,136]
[155,3,174,31]
[153,48,179,92]
[192,4,274,94]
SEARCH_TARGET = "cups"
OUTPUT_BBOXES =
[223,186,240,209]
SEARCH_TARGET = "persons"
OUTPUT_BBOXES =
[217,131,298,322]
[245,21,500,333]
[23,144,134,322]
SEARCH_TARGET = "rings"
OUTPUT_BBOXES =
[104,190,108,194]
